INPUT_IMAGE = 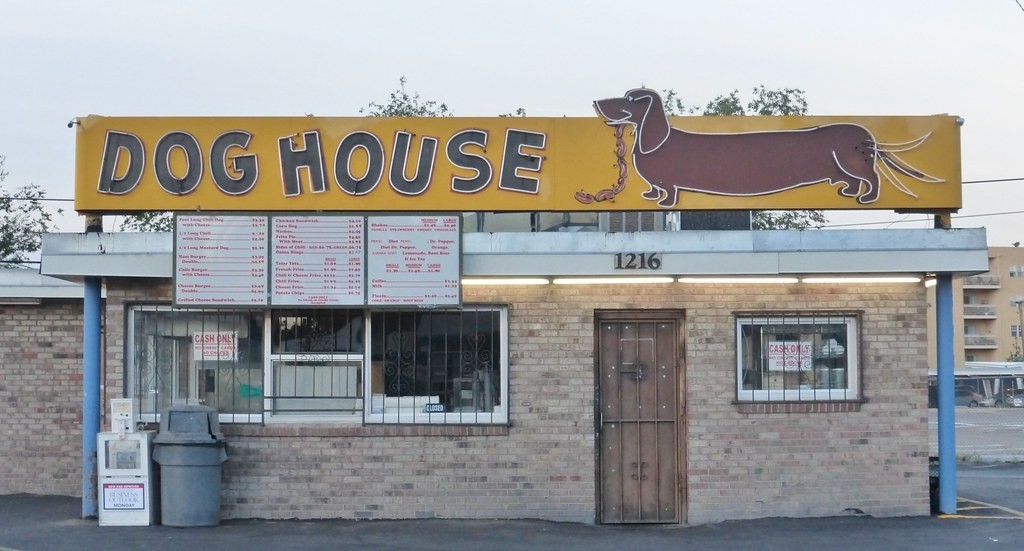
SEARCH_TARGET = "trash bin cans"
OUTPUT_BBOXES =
[151,405,231,528]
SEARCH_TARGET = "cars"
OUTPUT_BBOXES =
[954,386,1024,408]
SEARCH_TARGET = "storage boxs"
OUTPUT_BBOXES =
[801,333,821,356]
[800,369,820,389]
[763,373,784,390]
